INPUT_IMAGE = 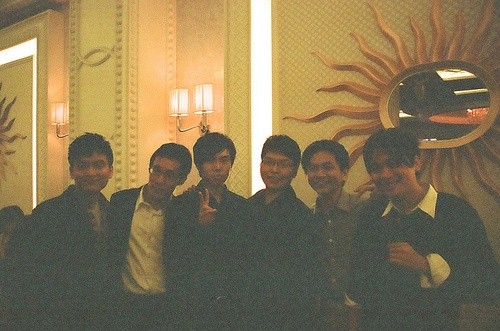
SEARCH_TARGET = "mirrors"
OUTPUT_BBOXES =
[378,61,500,150]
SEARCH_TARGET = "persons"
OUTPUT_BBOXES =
[171,133,250,330]
[301,140,375,331]
[109,143,195,330]
[242,135,315,330]
[2,206,23,264]
[32,134,120,331]
[349,127,493,330]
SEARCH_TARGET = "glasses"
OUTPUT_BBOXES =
[150,166,180,182]
[369,158,405,173]
[262,159,296,168]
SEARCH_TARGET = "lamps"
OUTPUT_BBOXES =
[170,83,213,136]
[48,101,70,138]
